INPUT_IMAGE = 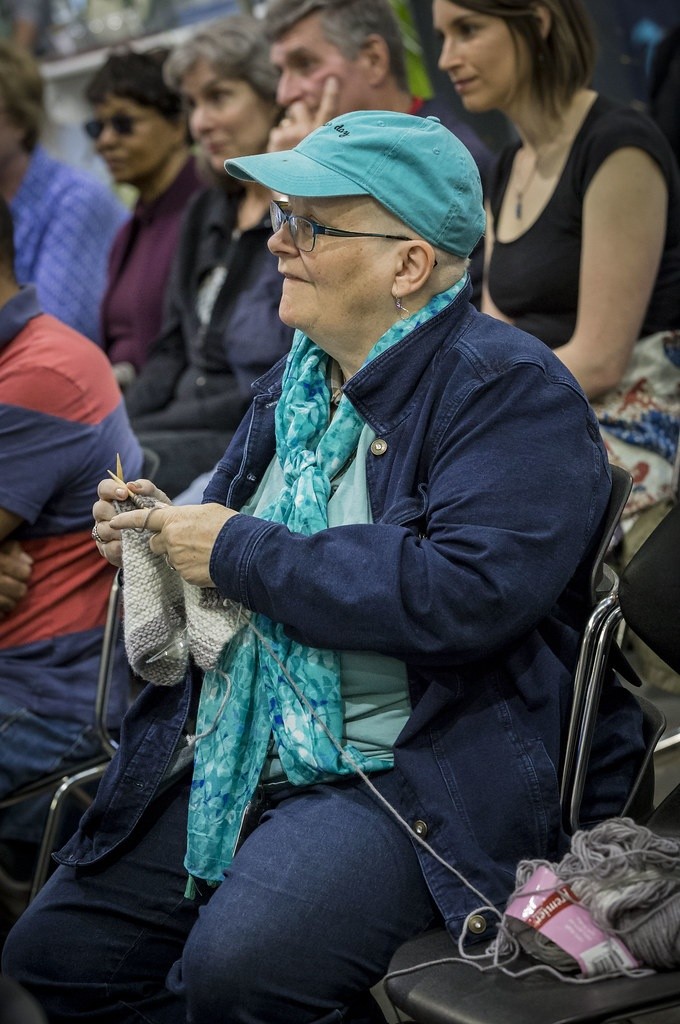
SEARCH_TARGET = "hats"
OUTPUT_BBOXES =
[224,111,487,260]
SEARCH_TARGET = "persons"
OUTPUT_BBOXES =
[431,1,680,663]
[124,14,289,497]
[230,0,489,403]
[0,41,132,351]
[0,110,655,1023]
[0,201,148,843]
[81,44,209,391]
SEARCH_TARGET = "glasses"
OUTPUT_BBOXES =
[85,113,156,138]
[269,200,439,269]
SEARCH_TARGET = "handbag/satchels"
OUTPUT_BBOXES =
[585,330,680,520]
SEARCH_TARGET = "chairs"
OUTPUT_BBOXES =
[378,460,680,1024]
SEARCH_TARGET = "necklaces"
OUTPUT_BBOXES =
[513,131,556,218]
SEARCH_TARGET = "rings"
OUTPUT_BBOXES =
[169,566,175,571]
[91,524,107,544]
